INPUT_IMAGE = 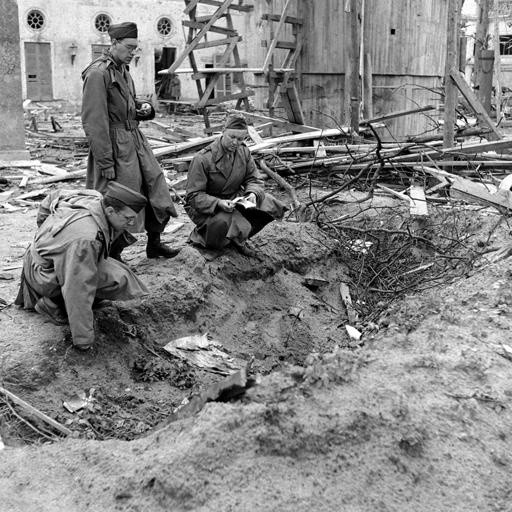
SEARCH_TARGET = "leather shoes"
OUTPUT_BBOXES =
[112,255,137,272]
[146,242,183,259]
[236,242,255,258]
[33,296,69,327]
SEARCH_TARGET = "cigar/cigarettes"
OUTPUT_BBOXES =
[143,108,147,114]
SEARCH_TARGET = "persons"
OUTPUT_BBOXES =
[78,22,183,265]
[9,180,152,352]
[182,113,288,258]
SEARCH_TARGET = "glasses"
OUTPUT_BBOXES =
[116,40,137,54]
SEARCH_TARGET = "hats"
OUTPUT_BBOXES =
[225,113,247,129]
[108,21,137,39]
[104,180,147,214]
